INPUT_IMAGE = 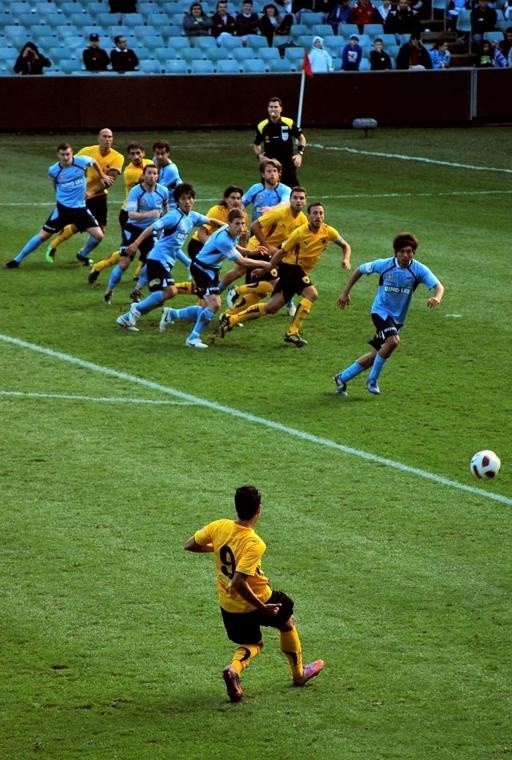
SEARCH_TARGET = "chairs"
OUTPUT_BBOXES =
[0,0,512,77]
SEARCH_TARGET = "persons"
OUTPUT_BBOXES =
[184,485,325,703]
[369,38,393,71]
[446,1,511,69]
[5,142,115,269]
[47,128,124,263]
[428,39,451,69]
[14,42,51,75]
[304,36,333,72]
[252,97,306,189]
[340,33,362,71]
[333,232,445,398]
[183,1,284,47]
[90,140,351,347]
[287,1,432,35]
[110,35,139,74]
[83,33,111,71]
[395,31,432,69]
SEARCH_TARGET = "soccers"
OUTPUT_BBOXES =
[470,450,500,479]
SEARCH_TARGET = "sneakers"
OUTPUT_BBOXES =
[77,251,93,266]
[223,667,242,703]
[284,333,308,345]
[334,374,348,397]
[287,300,297,317]
[186,285,243,348]
[88,264,170,332]
[7,260,19,268]
[46,243,56,263]
[293,659,324,685]
[367,379,381,394]
